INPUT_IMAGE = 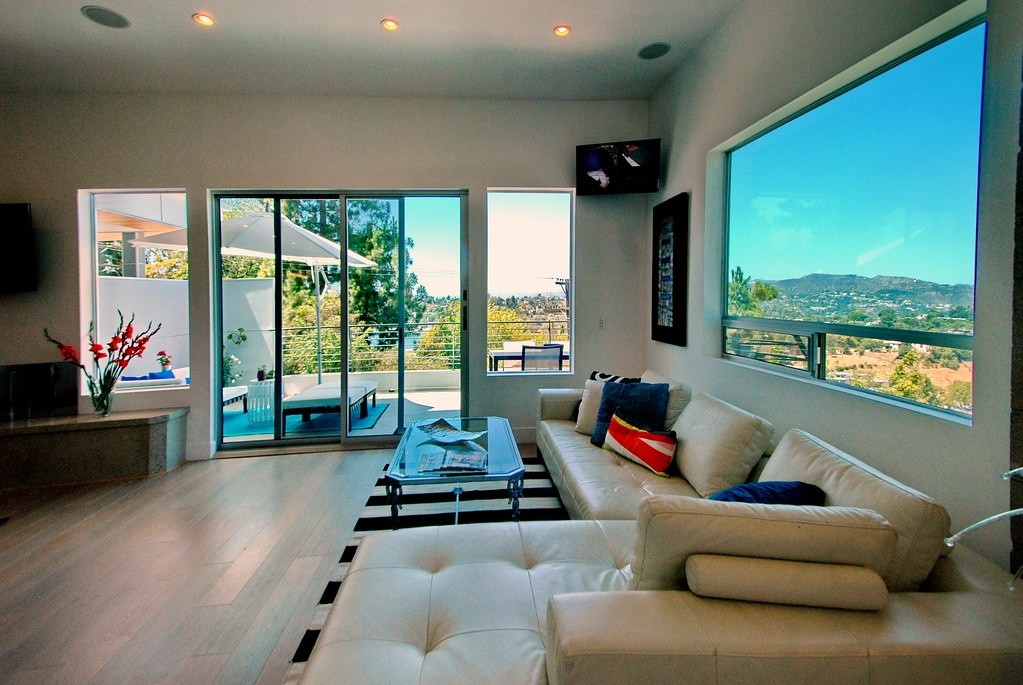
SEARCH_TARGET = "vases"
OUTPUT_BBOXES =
[162,364,171,372]
[86,377,117,417]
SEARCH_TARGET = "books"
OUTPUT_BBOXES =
[418,449,487,472]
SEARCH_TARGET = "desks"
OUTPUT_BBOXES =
[488,349,570,372]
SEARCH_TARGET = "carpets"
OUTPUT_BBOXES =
[223,403,389,438]
[279,458,571,685]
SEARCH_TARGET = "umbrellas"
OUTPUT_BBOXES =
[126,199,379,384]
[98,222,150,234]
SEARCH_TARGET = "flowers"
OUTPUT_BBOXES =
[155,350,173,365]
[43,308,161,414]
[223,345,246,387]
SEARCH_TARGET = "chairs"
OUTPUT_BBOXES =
[501,339,571,371]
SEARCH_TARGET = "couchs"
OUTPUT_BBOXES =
[297,369,1023,685]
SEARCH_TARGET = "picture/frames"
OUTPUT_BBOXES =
[650,191,691,348]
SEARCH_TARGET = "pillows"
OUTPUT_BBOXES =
[569,370,641,423]
[601,407,679,480]
[707,481,826,507]
[574,379,605,435]
[590,381,669,448]
[121,369,176,381]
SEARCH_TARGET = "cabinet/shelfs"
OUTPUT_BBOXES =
[247,378,275,427]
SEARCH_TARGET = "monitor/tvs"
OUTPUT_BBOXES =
[575,138,661,197]
[0,202,39,296]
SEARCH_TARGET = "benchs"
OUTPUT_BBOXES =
[115,366,248,414]
[282,380,377,437]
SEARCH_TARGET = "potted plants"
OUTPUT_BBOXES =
[256,365,267,381]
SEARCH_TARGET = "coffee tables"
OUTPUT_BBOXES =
[385,416,525,530]
